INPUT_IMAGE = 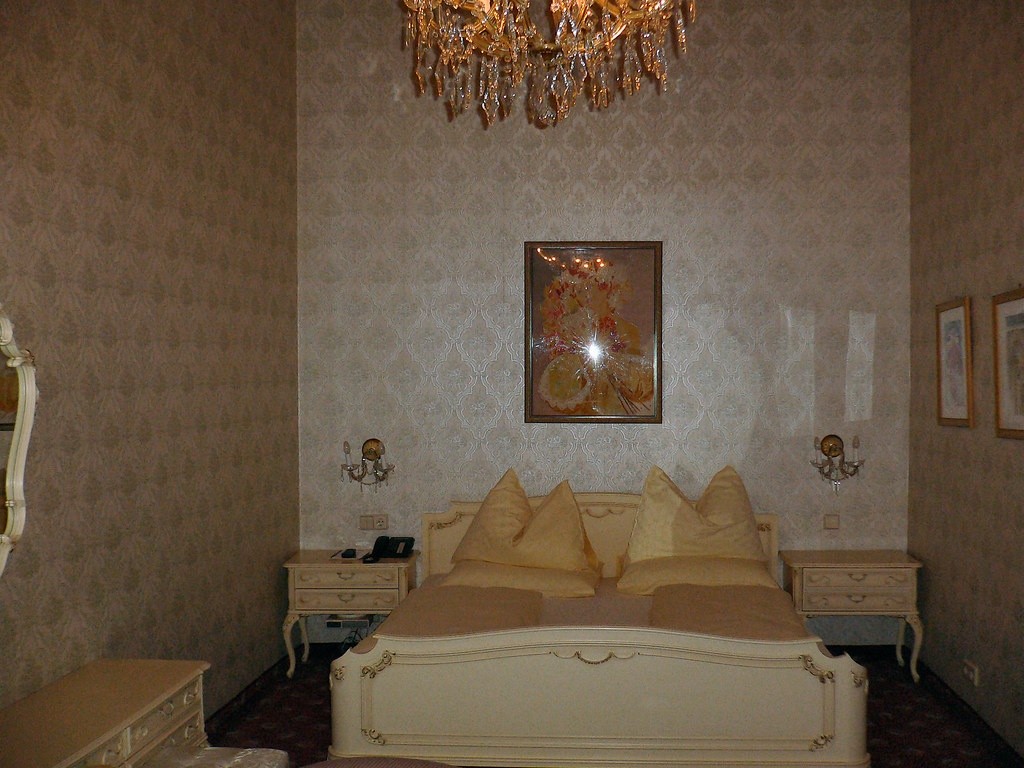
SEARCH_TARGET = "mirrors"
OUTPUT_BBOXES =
[0,294,40,590]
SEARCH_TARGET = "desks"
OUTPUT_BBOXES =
[0,653,212,768]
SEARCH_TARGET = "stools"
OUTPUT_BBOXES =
[138,744,291,768]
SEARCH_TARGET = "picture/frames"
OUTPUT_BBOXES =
[525,241,662,424]
[934,295,975,427]
[991,288,1024,440]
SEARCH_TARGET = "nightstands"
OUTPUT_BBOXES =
[774,541,932,682]
[282,550,420,678]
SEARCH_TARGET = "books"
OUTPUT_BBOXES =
[331,550,369,560]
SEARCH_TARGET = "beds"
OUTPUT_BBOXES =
[325,496,875,768]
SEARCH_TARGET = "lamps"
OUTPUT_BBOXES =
[811,433,865,484]
[339,437,398,492]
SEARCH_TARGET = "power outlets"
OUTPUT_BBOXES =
[374,516,389,530]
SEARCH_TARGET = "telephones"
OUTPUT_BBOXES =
[373,536,416,557]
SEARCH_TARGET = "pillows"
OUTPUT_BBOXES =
[616,462,783,598]
[449,470,607,599]
